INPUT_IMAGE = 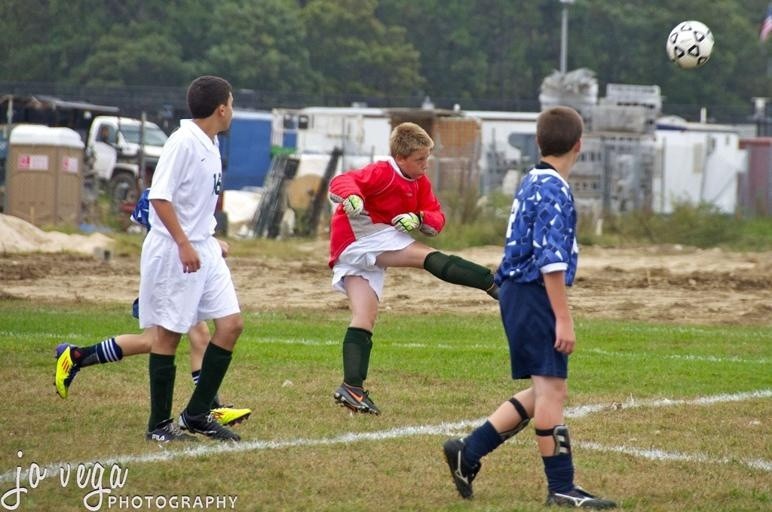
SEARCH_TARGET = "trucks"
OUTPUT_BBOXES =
[0,95,172,206]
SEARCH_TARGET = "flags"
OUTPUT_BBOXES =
[759,3,772,41]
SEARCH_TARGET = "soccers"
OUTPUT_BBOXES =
[667,21,715,68]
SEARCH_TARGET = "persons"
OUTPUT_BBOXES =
[443,105,618,511]
[100,127,109,143]
[327,122,500,417]
[138,75,245,444]
[53,185,253,427]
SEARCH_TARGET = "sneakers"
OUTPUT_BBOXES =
[178,408,241,444]
[443,438,481,502]
[54,344,80,400]
[545,484,618,509]
[334,381,381,416]
[209,405,252,427]
[145,418,180,444]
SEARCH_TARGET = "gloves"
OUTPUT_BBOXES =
[343,195,364,219]
[391,211,421,233]
